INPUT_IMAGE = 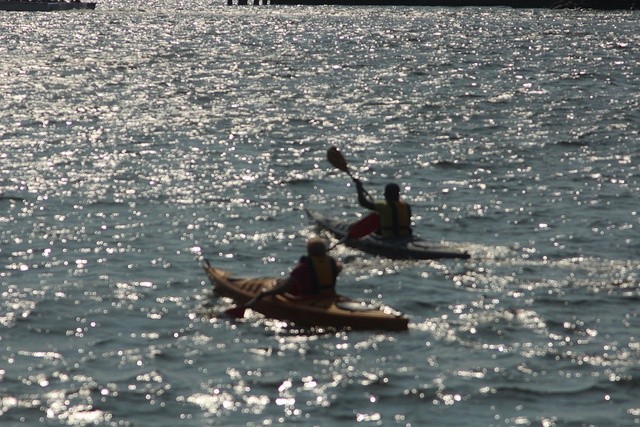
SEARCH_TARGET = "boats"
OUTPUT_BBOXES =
[199,257,411,332]
[302,205,474,261]
[0,0,97,11]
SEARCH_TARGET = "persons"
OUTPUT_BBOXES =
[259,235,343,302]
[356,178,412,245]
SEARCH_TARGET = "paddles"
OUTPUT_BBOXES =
[223,210,381,318]
[327,147,375,202]
